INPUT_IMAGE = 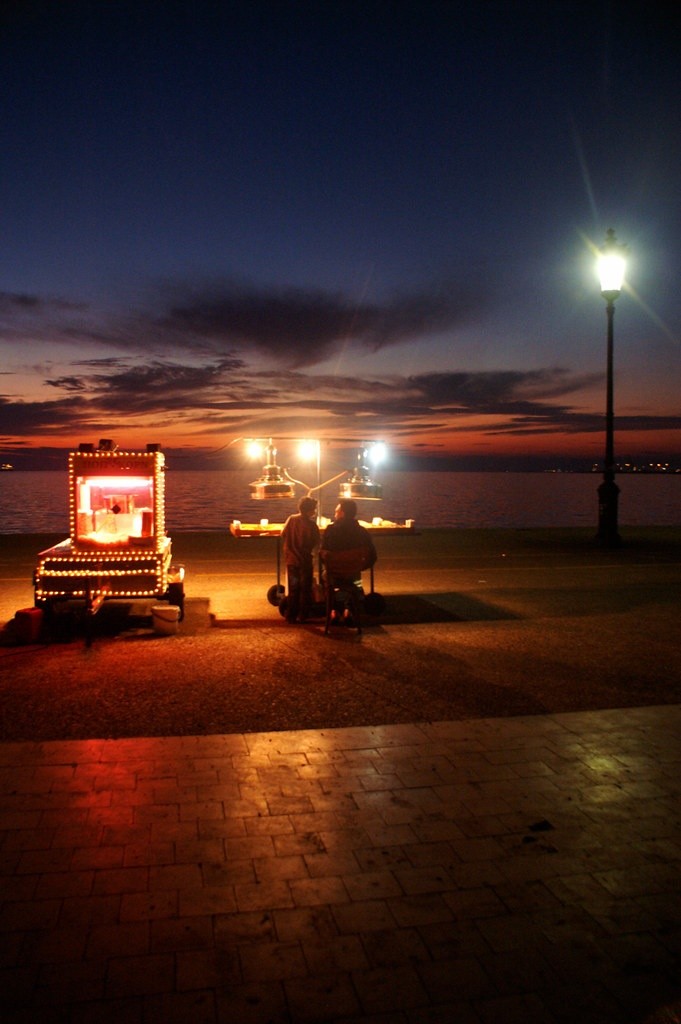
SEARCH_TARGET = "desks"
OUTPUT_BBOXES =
[229,517,415,618]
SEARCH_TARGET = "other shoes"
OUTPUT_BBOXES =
[330,609,341,625]
[342,611,353,625]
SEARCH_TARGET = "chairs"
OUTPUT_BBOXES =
[318,545,375,638]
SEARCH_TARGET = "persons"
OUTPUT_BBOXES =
[280,496,320,624]
[317,500,377,625]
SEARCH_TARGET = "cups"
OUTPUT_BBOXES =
[261,519,268,529]
[234,520,241,529]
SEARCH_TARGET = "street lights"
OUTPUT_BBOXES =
[596,248,629,550]
